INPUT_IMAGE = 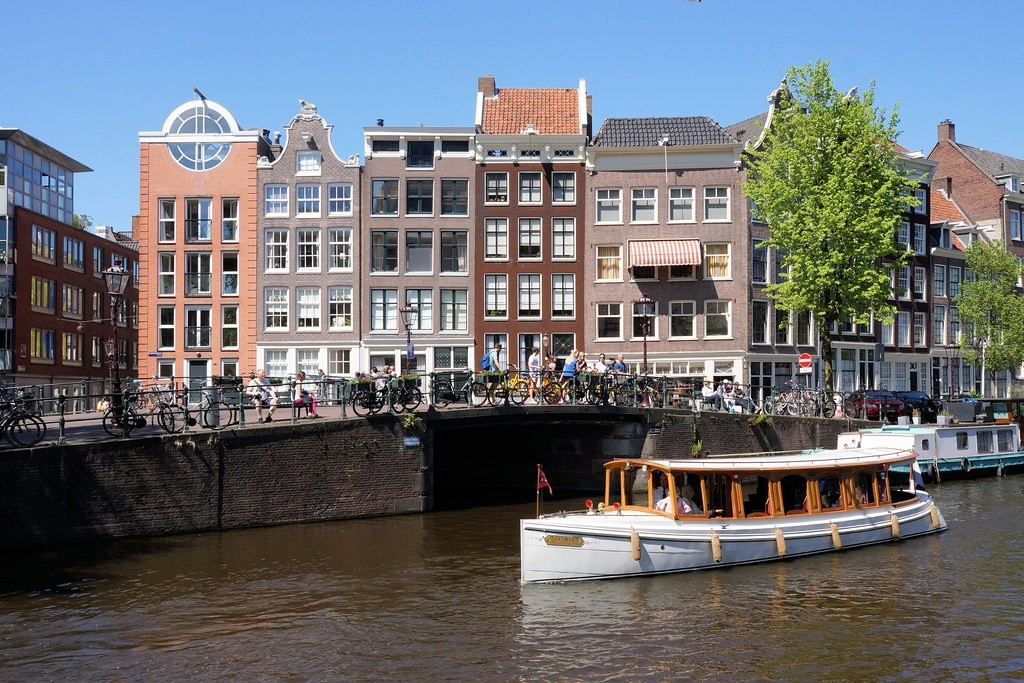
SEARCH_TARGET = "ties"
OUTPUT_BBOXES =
[662,489,666,498]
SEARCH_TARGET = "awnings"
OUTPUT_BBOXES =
[628,240,701,266]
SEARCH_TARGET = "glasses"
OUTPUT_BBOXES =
[600,357,604,358]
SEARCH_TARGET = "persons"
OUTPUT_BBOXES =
[291,371,324,418]
[558,350,587,405]
[700,379,761,414]
[654,473,723,517]
[246,369,280,424]
[485,343,502,405]
[594,352,626,406]
[370,365,397,378]
[96,396,109,413]
[317,369,325,380]
[528,346,557,405]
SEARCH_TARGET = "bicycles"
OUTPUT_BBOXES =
[764,380,837,419]
[488,363,634,406]
[352,377,422,418]
[429,368,489,409]
[0,381,47,449]
[101,373,233,437]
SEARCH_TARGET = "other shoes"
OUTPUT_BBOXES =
[258,417,264,425]
[754,405,762,414]
[266,416,276,422]
[308,412,324,418]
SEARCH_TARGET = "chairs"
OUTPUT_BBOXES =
[786,509,807,515]
[747,512,770,518]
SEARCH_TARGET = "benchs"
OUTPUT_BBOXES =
[698,401,744,413]
[224,393,311,422]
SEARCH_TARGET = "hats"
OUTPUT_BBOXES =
[609,356,615,360]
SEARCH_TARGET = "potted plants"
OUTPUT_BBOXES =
[345,377,375,392]
[267,377,282,386]
[477,369,508,382]
[912,408,922,424]
[392,374,421,388]
[580,371,605,383]
[937,410,950,426]
[211,374,243,385]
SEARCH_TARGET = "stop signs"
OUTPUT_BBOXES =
[799,352,812,368]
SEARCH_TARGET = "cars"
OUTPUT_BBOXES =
[845,390,905,419]
[892,389,939,423]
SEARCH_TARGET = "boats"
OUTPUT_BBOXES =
[519,445,948,584]
[835,422,1024,486]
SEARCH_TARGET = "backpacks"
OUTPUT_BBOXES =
[480,351,495,371]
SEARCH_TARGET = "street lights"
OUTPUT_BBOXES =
[102,257,133,430]
[632,292,657,407]
[398,303,419,379]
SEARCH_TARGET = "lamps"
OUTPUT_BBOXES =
[613,502,621,515]
[585,500,594,516]
